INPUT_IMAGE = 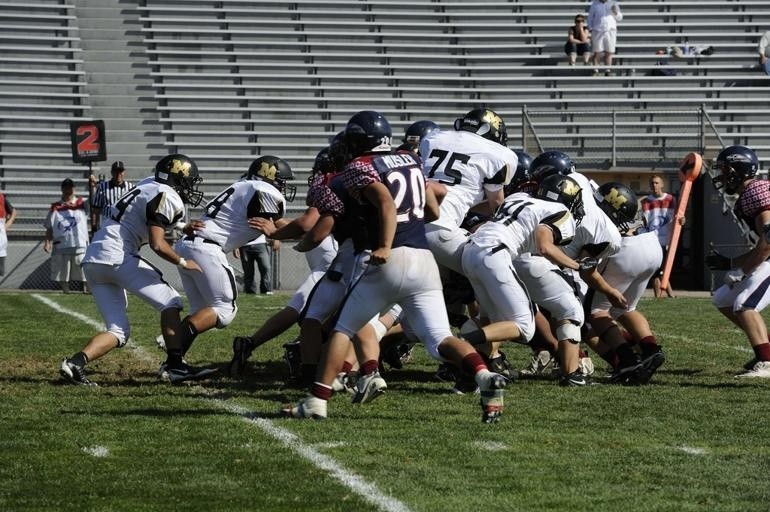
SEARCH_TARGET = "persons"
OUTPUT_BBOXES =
[758,31,770,76]
[228,109,664,424]
[705,146,770,379]
[155,154,297,383]
[59,155,205,388]
[637,174,686,300]
[587,0,623,75]
[564,15,592,65]
[43,162,137,295]
[0,190,17,258]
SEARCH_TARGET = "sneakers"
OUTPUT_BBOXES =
[229,336,254,381]
[435,349,601,396]
[331,371,359,393]
[733,358,770,381]
[58,358,100,386]
[479,371,506,424]
[353,370,388,404]
[384,344,416,369]
[156,332,218,385]
[280,392,330,420]
[602,342,666,387]
[283,340,306,383]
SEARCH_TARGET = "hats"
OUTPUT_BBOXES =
[111,160,126,173]
[60,178,77,189]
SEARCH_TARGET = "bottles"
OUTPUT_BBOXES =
[665,41,696,56]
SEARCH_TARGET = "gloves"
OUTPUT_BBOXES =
[724,267,750,290]
[704,248,734,272]
[577,255,603,272]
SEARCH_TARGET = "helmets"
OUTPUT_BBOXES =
[344,110,393,147]
[593,182,639,232]
[154,153,204,207]
[535,175,586,228]
[307,130,352,186]
[712,145,758,194]
[247,155,296,203]
[505,150,575,193]
[455,108,508,147]
[401,121,441,147]
